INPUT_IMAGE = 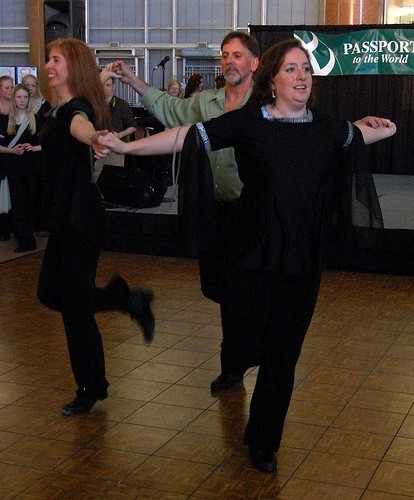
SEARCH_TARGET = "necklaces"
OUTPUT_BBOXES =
[275,106,307,119]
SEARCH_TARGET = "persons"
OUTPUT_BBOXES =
[1,75,15,240]
[22,74,51,236]
[158,81,181,184]
[103,74,138,142]
[112,32,393,391]
[98,40,396,473]
[184,75,204,99]
[1,84,44,252]
[36,39,156,415]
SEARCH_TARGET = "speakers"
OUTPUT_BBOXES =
[97,164,167,209]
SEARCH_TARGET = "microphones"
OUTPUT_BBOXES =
[154,56,170,70]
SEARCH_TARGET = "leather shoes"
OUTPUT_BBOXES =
[248,439,277,473]
[130,288,156,341]
[211,371,244,393]
[64,390,109,415]
[14,244,37,252]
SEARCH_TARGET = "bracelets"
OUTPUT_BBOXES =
[129,77,141,88]
